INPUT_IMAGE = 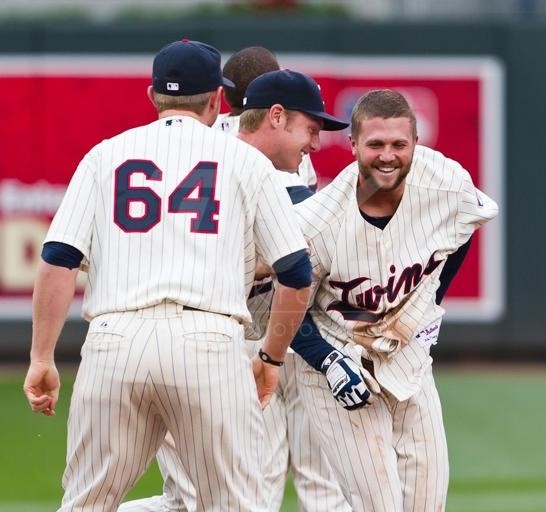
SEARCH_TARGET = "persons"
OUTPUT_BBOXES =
[119,47,353,509]
[23,40,313,512]
[291,88,500,511]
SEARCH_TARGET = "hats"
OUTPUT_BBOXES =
[241,69,349,131]
[151,38,236,96]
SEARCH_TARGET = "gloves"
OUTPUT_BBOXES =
[319,349,381,411]
